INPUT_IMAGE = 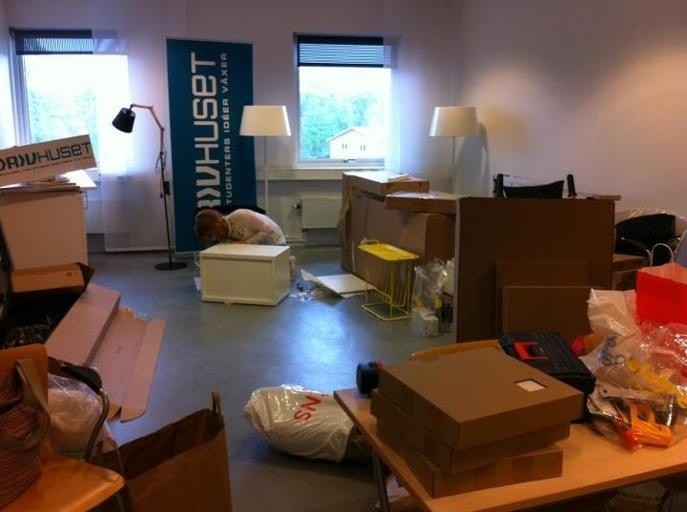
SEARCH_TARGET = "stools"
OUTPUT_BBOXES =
[357,239,421,321]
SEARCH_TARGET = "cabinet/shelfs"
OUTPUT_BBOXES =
[199,242,291,306]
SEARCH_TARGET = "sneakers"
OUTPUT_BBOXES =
[289,256,297,280]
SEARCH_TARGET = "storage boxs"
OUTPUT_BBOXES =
[342,170,458,309]
[0,167,166,423]
[369,341,584,499]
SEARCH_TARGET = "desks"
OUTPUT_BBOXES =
[333,388,687,512]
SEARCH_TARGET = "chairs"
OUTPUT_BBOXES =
[1,344,126,512]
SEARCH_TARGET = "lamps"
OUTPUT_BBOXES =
[430,105,478,194]
[240,105,292,218]
[112,104,188,271]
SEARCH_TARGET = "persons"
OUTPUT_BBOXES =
[195,208,287,248]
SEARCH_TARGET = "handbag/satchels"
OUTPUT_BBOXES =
[614,213,687,386]
[89,391,233,512]
[0,358,50,509]
